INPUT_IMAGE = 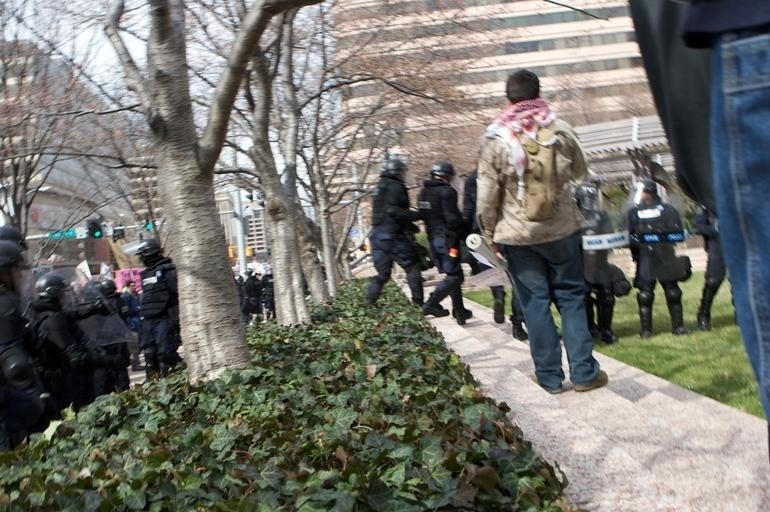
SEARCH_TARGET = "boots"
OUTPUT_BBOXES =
[584,299,618,345]
[697,286,719,331]
[667,301,691,335]
[639,304,653,339]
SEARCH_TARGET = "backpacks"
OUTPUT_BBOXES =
[503,120,564,222]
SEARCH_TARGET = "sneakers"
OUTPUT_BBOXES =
[494,296,505,323]
[573,370,609,392]
[422,298,450,318]
[512,325,529,341]
[530,370,564,394]
[452,307,473,325]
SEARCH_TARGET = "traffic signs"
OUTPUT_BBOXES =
[47,228,75,240]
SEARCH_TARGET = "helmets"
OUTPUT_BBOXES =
[429,160,457,178]
[35,274,71,298]
[135,238,162,261]
[235,267,254,286]
[100,280,117,295]
[0,225,29,271]
[385,159,408,176]
[632,178,657,196]
[574,183,598,201]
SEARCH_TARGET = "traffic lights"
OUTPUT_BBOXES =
[255,185,264,208]
[143,220,155,233]
[246,182,252,202]
[86,218,102,238]
